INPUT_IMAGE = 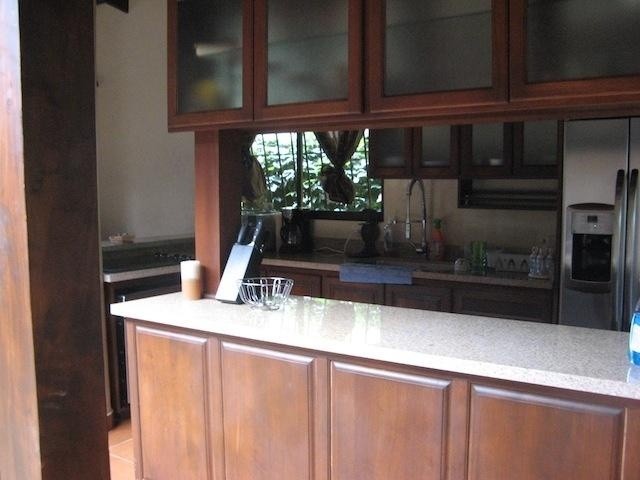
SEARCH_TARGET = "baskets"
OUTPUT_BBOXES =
[237,277,294,312]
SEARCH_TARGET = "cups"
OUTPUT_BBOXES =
[179,258,204,302]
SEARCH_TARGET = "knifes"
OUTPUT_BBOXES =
[235,215,265,247]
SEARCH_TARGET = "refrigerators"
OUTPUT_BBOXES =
[558,116,640,333]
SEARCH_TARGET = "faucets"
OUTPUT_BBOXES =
[402,173,428,255]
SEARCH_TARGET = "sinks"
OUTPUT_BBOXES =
[350,258,423,273]
[415,262,457,274]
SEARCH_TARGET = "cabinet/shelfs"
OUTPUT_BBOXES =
[324,277,452,313]
[364,121,564,181]
[257,269,322,298]
[455,287,554,324]
[365,0,639,112]
[167,1,360,127]
